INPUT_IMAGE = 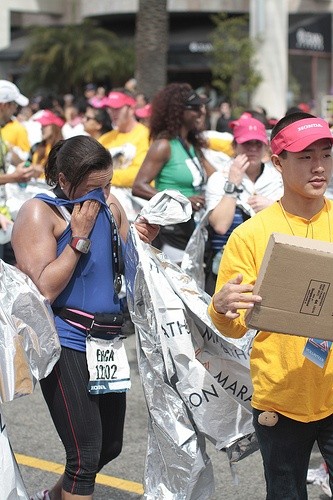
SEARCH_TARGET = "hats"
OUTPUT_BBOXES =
[232,118,269,147]
[33,109,65,127]
[0,78,30,107]
[228,112,252,128]
[100,91,136,110]
[181,90,211,105]
[271,117,333,155]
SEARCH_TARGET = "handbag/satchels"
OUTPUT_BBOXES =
[50,308,125,340]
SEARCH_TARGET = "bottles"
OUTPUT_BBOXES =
[17,161,31,188]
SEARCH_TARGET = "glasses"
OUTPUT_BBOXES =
[81,115,96,121]
[182,104,205,110]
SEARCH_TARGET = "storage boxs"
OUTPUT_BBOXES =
[244,232,333,343]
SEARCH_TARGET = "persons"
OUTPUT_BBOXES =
[10,135,163,500]
[0,76,333,299]
[207,114,333,500]
